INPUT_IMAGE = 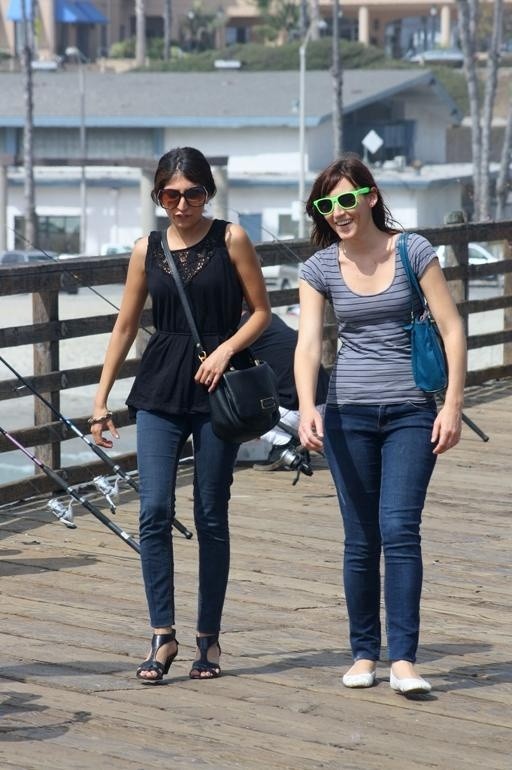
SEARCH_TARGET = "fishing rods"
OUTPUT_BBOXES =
[6,224,313,486]
[1,427,141,555]
[1,356,193,539]
[212,203,490,442]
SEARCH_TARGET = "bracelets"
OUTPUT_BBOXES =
[87,409,113,425]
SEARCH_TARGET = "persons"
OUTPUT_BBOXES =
[290,154,471,697]
[89,144,274,685]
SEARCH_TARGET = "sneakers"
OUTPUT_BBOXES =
[252,435,310,471]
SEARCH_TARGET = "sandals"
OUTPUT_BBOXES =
[137,626,180,681]
[188,632,222,679]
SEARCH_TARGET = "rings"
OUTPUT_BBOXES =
[207,380,211,383]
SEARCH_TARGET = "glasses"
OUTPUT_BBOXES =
[158,185,209,210]
[313,186,372,215]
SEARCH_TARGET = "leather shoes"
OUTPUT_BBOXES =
[342,658,378,689]
[389,665,434,695]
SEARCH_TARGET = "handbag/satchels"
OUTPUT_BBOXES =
[398,231,449,395]
[207,358,281,444]
